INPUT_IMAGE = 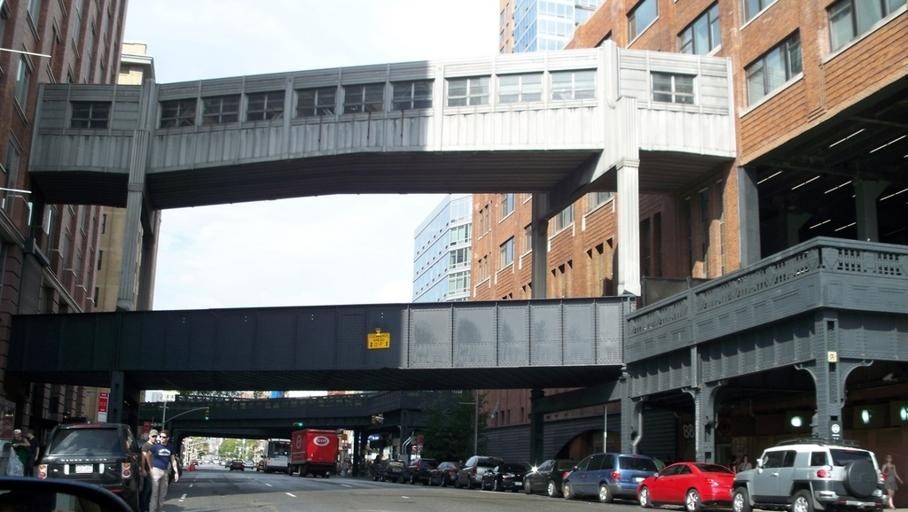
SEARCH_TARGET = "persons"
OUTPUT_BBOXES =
[881,455,903,512]
[25,430,40,477]
[8,429,30,477]
[147,431,179,512]
[140,429,158,512]
[730,455,751,473]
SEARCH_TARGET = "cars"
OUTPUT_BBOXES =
[1,420,183,512]
[191,454,253,471]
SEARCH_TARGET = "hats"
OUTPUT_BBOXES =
[149,429,159,436]
[14,429,22,434]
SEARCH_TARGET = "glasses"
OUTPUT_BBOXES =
[151,436,157,438]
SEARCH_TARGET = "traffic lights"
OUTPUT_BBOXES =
[205,411,209,420]
[294,422,303,426]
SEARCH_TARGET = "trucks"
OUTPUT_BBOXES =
[255,429,339,477]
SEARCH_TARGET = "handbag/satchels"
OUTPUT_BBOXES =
[6,447,25,477]
[883,473,888,480]
